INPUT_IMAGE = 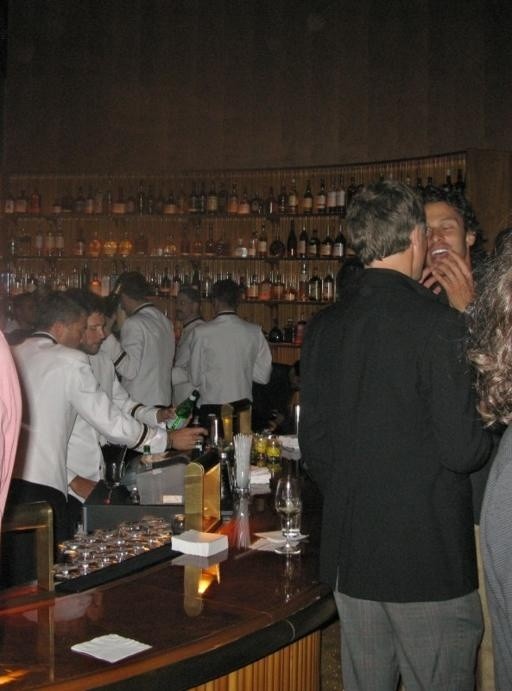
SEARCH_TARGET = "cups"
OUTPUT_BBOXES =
[226,459,251,493]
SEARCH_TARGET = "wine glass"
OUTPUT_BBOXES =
[276,476,303,555]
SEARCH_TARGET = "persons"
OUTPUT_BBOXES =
[291,183,511,690]
[1,270,274,587]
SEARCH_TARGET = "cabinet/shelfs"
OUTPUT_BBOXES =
[0,154,466,404]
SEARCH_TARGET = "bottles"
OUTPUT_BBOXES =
[167,390,200,433]
[130,487,139,505]
[141,445,151,465]
[2,169,466,343]
[172,514,185,534]
